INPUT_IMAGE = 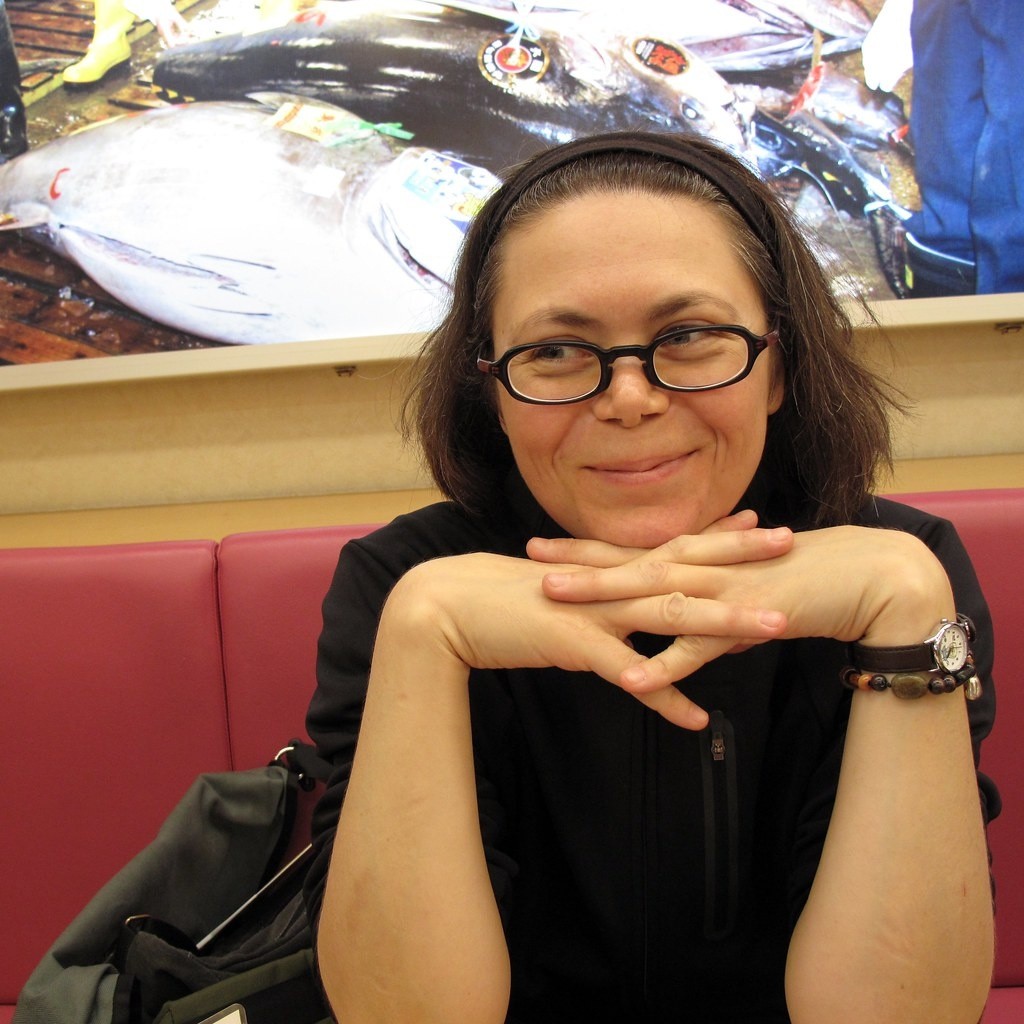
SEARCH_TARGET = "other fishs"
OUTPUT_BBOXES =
[0,0,911,343]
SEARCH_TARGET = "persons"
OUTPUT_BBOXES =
[305,131,1002,1024]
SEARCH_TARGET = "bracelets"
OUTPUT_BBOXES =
[842,665,982,702]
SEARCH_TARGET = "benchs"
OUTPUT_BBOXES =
[0,489,1024,1024]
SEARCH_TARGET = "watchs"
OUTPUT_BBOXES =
[846,614,978,677]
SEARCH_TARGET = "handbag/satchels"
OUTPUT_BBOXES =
[13,757,329,1024]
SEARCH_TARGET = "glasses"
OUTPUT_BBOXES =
[476,314,781,405]
